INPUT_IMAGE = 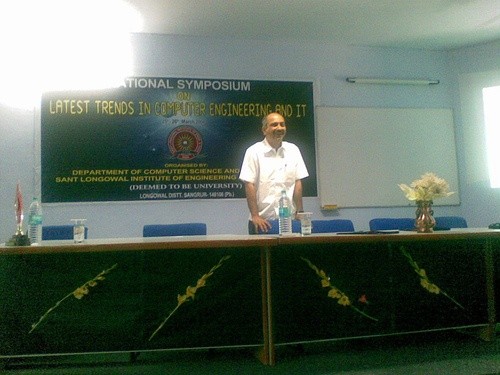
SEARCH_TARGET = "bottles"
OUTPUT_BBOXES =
[28,197,43,247]
[278,189,292,236]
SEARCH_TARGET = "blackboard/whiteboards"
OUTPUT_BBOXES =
[314,106,461,209]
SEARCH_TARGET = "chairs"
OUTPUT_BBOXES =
[311,219,355,233]
[433,216,468,228]
[248,220,301,234]
[369,218,416,231]
[143,223,206,236]
[26,225,88,240]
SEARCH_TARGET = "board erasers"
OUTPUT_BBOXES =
[323,205,337,210]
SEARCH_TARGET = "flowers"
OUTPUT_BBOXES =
[396,172,455,201]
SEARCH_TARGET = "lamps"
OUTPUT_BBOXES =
[346,77,440,86]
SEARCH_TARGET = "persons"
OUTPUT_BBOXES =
[238,112,310,235]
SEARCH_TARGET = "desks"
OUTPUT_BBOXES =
[0,227,500,371]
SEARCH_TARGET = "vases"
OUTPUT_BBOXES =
[414,202,435,233]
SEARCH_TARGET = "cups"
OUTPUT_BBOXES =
[297,212,313,235]
[71,219,87,244]
